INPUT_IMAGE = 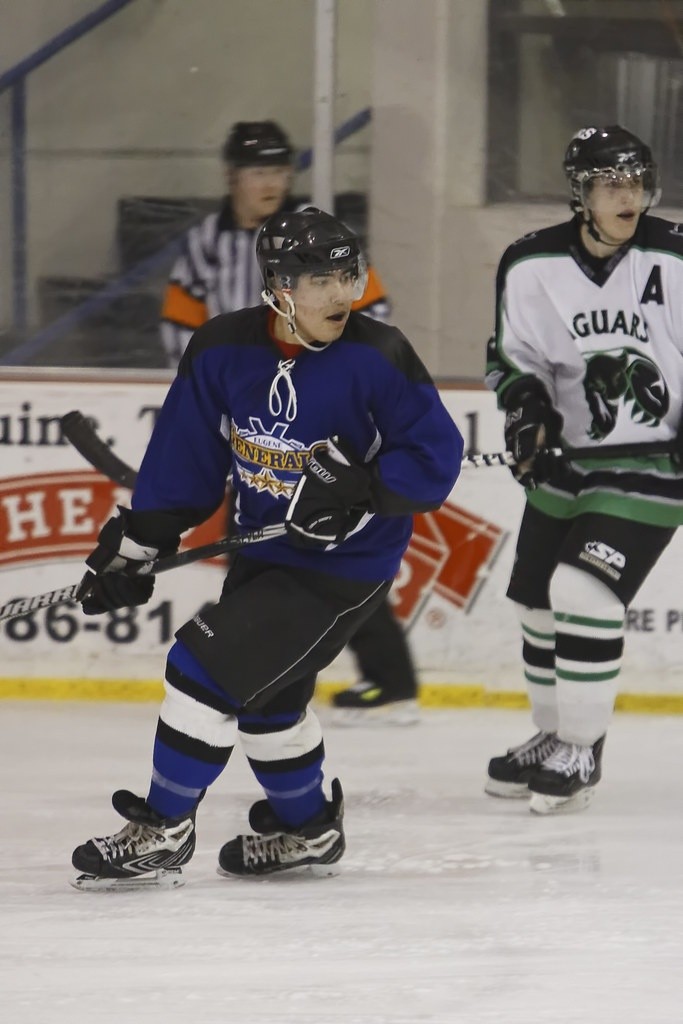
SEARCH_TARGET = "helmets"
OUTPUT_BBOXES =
[255,207,361,274]
[223,120,293,165]
[562,124,653,183]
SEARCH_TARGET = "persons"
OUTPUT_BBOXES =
[158,117,421,725]
[483,124,683,817]
[68,205,465,893]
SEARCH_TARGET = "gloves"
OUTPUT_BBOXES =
[284,451,377,553]
[499,375,564,492]
[73,504,188,616]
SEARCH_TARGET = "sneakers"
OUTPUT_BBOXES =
[214,777,346,880]
[527,735,607,814]
[481,730,560,797]
[326,679,422,726]
[69,787,207,892]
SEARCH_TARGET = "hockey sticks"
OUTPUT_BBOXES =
[0,521,291,625]
[60,405,681,492]
